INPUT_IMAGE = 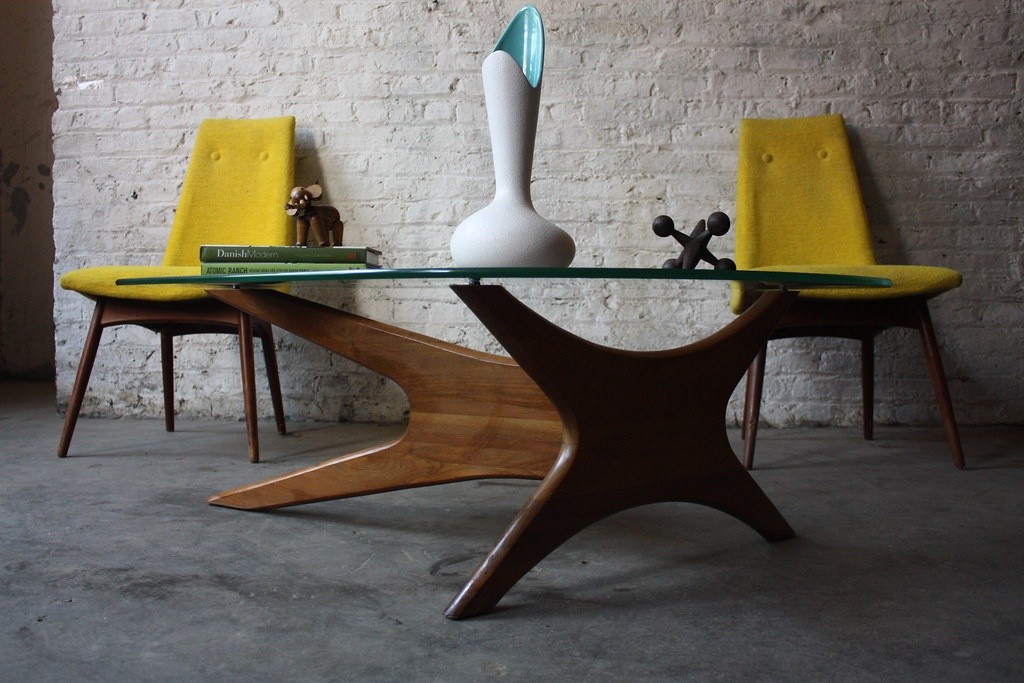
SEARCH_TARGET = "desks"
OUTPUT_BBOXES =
[116,268,897,623]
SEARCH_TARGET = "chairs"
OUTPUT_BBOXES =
[56,116,297,464]
[730,113,968,474]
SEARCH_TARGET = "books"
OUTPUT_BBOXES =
[198,244,383,276]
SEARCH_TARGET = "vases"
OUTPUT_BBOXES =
[448,6,578,268]
